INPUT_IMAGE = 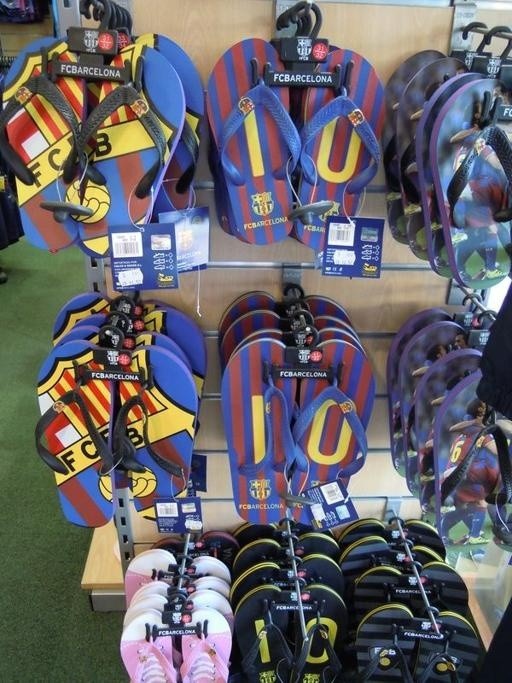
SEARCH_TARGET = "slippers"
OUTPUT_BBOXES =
[120,609,177,683]
[3,28,203,259]
[287,584,351,683]
[339,517,469,653]
[235,584,295,683]
[179,605,233,683]
[381,49,511,291]
[217,290,377,527]
[32,290,215,532]
[355,603,417,683]
[203,38,388,251]
[123,530,239,637]
[413,610,482,683]
[387,304,483,517]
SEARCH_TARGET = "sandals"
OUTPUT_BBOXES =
[229,518,345,614]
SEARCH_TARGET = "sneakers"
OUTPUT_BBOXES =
[439,531,493,548]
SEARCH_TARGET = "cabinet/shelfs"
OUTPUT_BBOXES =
[79,0,512,615]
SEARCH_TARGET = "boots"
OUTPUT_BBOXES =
[432,367,512,556]
